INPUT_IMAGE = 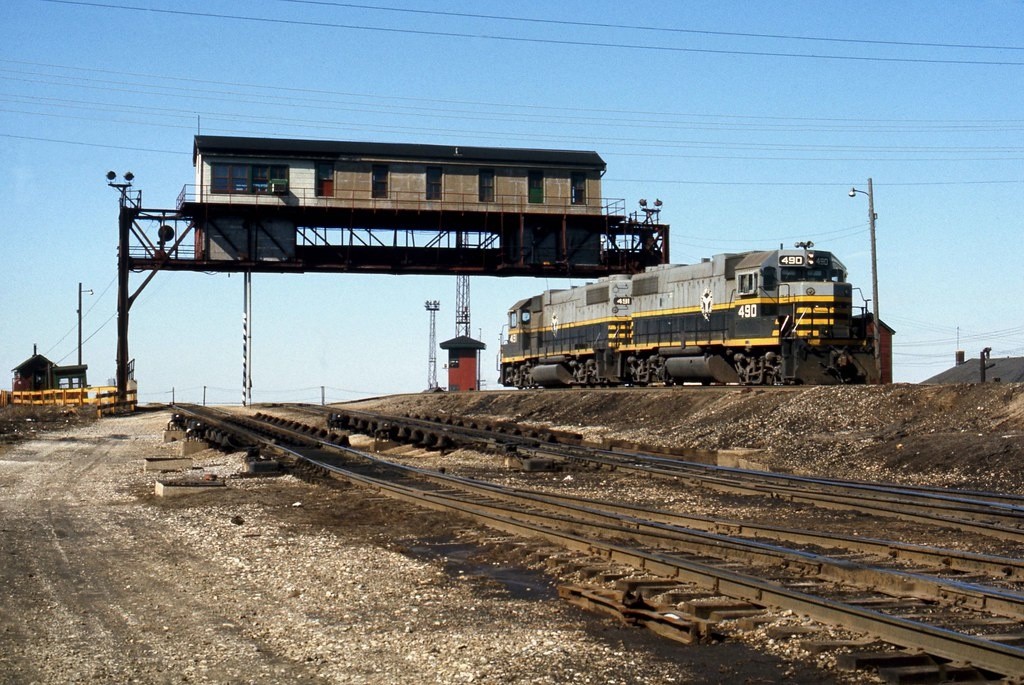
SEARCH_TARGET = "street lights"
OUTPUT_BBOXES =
[848,178,883,384]
[77,282,94,387]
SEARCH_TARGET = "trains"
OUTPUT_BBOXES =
[496,240,872,391]
